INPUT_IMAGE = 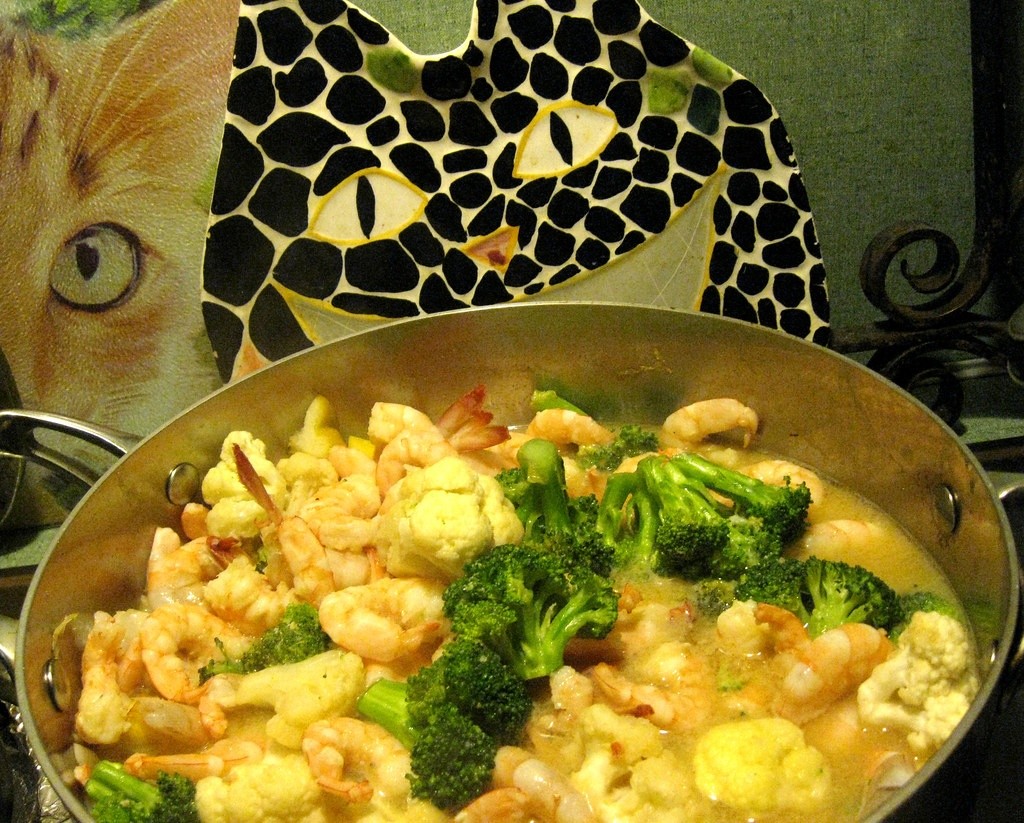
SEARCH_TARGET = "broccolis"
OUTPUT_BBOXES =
[78,386,976,823]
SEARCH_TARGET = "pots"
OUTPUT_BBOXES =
[0,301,1024,823]
